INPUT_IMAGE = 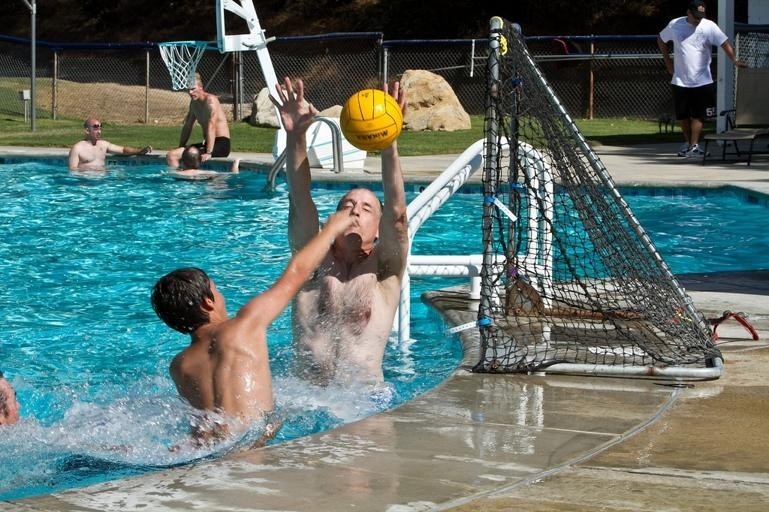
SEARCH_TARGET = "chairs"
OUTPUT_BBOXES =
[700,63,768,167]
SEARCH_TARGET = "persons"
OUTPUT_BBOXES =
[176,146,240,176]
[0,372,21,427]
[267,76,409,383]
[656,1,749,158]
[166,73,230,168]
[149,203,361,446]
[68,118,153,172]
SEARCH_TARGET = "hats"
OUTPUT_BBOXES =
[689,0,706,18]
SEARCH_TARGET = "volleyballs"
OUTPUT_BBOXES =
[340,89,404,152]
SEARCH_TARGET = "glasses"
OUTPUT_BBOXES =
[87,123,104,129]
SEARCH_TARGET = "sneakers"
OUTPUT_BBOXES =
[677,144,710,158]
